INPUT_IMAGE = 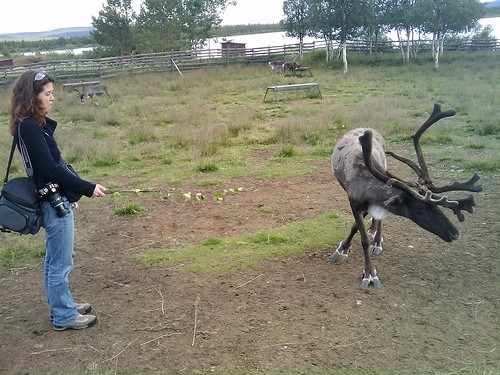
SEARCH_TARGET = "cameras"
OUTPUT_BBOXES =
[38,182,70,218]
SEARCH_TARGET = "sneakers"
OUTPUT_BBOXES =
[50,303,91,322]
[54,315,96,331]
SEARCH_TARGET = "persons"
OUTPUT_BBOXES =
[10,69,106,332]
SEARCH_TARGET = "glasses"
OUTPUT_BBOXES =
[31,70,48,83]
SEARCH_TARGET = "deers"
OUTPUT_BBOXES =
[331,103,482,290]
[269,59,313,79]
[74,81,114,107]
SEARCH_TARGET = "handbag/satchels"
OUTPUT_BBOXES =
[0,177,43,235]
